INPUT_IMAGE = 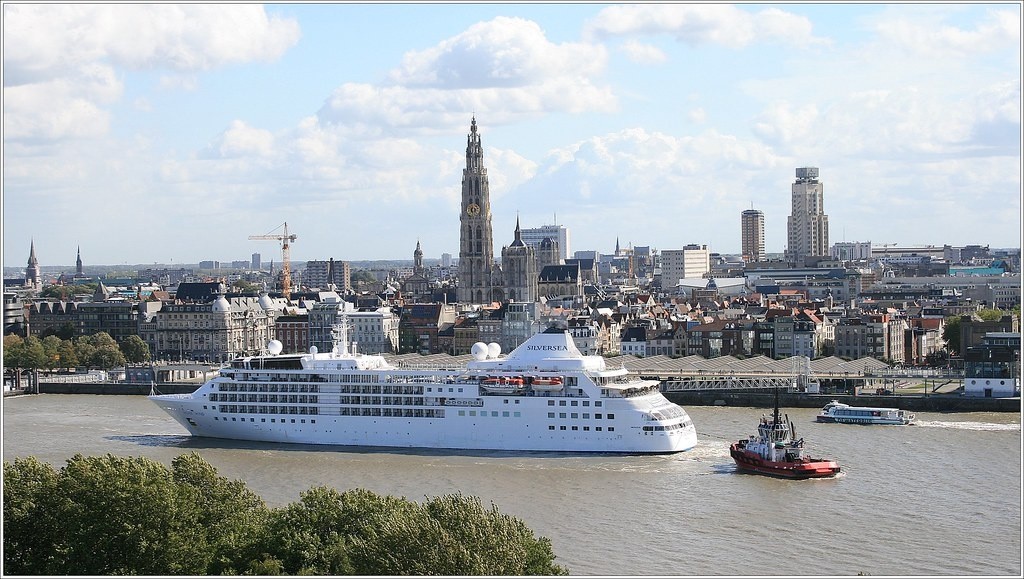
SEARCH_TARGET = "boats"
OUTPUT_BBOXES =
[816,400,915,425]
[728,384,841,478]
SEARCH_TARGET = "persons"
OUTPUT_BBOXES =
[876,388,884,395]
[801,385,805,392]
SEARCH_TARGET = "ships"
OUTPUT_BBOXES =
[149,327,698,453]
